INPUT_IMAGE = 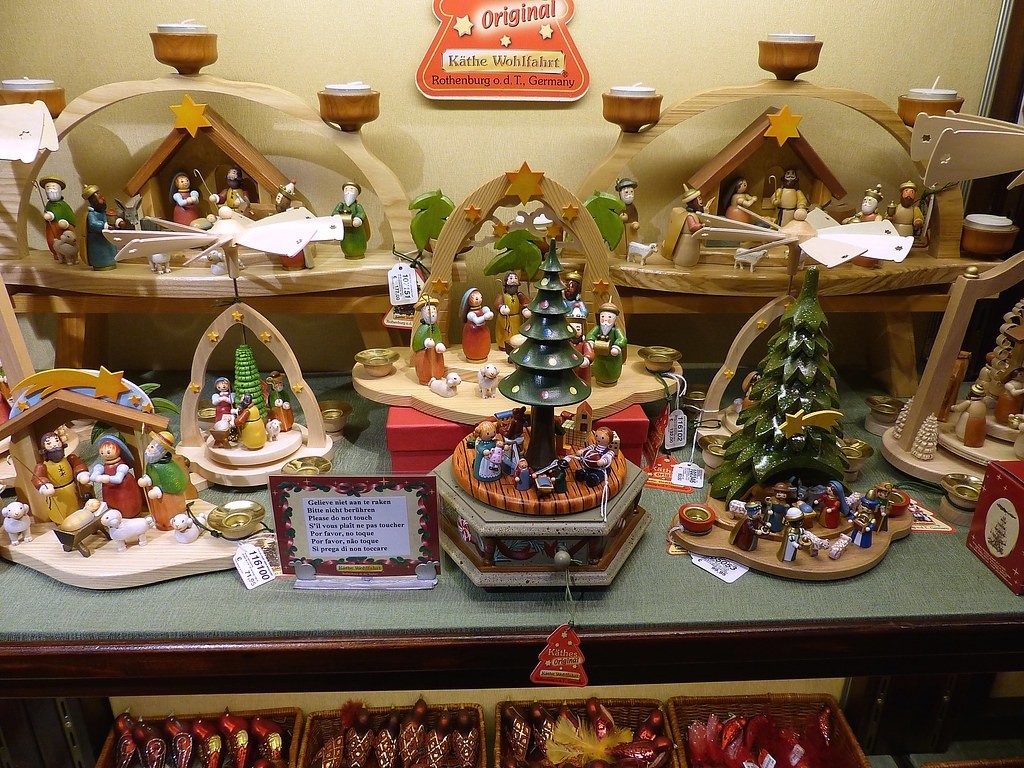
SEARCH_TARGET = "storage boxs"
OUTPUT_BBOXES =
[966,461,1024,597]
[386,406,649,473]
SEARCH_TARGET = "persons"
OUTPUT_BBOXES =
[660,183,707,268]
[31,431,185,531]
[493,271,532,350]
[951,352,995,448]
[212,371,292,449]
[615,178,641,258]
[81,184,126,271]
[771,167,807,227]
[549,459,570,493]
[332,181,372,260]
[513,459,537,491]
[274,179,314,271]
[576,427,615,487]
[564,272,627,387]
[38,176,76,260]
[841,181,924,267]
[995,369,1024,422]
[730,481,893,561]
[459,288,493,363]
[723,177,757,224]
[414,295,446,385]
[473,422,509,482]
[168,166,251,270]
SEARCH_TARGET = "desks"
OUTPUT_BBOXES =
[0,365,1023,768]
